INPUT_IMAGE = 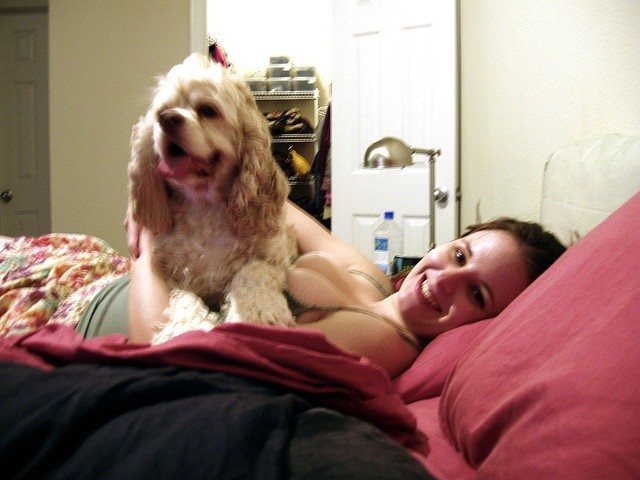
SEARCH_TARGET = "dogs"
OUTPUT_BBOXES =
[127,54,299,348]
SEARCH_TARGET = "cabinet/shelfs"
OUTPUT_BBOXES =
[251,88,322,201]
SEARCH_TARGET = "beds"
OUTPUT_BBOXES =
[0,121,640,480]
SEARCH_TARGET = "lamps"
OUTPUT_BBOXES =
[363,136,437,276]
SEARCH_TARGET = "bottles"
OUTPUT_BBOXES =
[374,211,403,277]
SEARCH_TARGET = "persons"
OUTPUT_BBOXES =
[70,200,568,377]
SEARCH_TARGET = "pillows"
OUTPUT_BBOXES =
[390,316,497,405]
[436,190,639,479]
